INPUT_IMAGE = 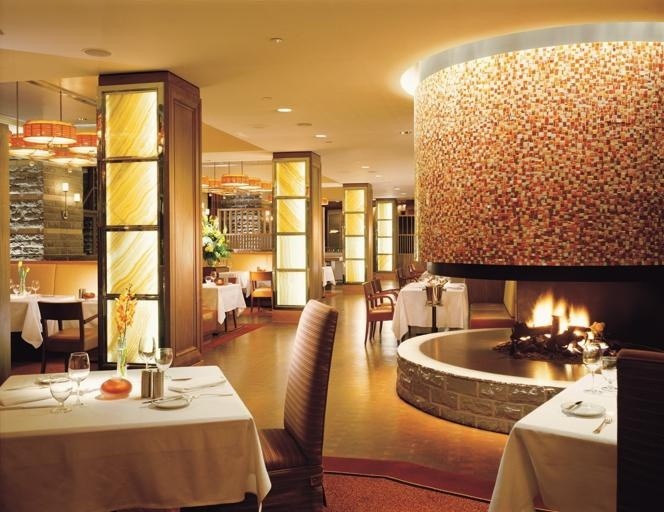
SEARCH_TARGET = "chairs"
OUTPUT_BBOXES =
[250,271,275,311]
[233,298,339,510]
[37,300,98,373]
[616,348,663,512]
[361,263,425,346]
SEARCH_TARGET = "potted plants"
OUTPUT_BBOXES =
[197,207,229,282]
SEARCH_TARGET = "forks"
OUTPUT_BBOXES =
[592,417,611,434]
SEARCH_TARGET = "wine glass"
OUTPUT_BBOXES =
[206,271,217,284]
[68,352,91,408]
[139,336,156,369]
[10,279,41,295]
[50,375,72,414]
[583,344,618,393]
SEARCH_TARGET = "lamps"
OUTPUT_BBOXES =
[199,162,273,222]
[4,78,100,220]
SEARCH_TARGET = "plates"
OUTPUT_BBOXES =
[561,401,606,417]
[153,396,190,408]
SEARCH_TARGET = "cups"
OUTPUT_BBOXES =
[153,348,174,371]
